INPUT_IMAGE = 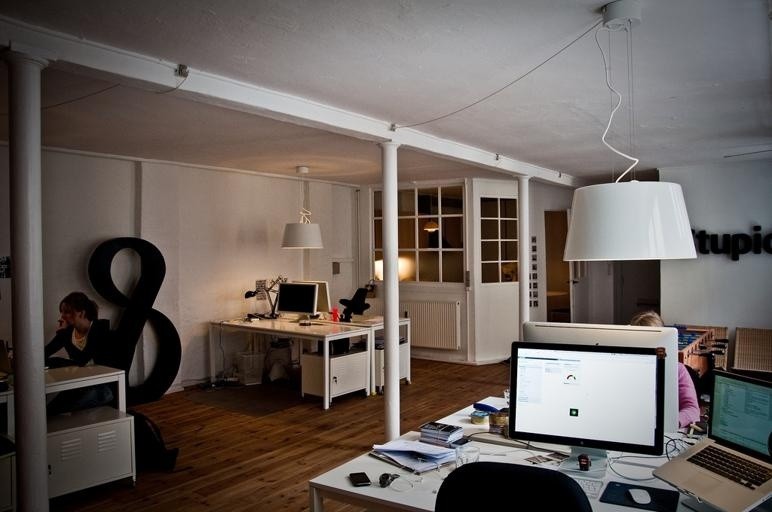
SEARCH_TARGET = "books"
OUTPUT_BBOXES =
[419,420,469,449]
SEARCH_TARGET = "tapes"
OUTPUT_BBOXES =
[471,411,489,424]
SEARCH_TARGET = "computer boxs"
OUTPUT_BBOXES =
[318,340,350,358]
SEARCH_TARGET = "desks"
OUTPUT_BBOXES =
[307,428,771,512]
[14,366,136,507]
[208,313,375,408]
[341,309,417,397]
[434,393,697,467]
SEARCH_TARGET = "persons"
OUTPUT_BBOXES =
[46,290,113,405]
[629,309,701,430]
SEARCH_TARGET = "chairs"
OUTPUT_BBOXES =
[688,322,735,369]
[433,461,595,511]
[731,327,772,372]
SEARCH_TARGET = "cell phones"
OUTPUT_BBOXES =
[349,471,371,487]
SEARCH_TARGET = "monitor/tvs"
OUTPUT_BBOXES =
[508,341,666,479]
[522,320,679,435]
[278,280,332,323]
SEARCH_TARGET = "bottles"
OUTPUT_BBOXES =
[331,302,340,322]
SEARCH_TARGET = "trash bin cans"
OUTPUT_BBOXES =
[236,352,265,386]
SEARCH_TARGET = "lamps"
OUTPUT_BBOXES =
[560,0,700,261]
[280,166,325,248]
[245,286,284,320]
[423,195,439,233]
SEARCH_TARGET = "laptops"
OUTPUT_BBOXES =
[652,368,772,512]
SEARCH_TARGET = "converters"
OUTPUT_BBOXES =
[451,437,471,448]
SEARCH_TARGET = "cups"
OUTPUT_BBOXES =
[455,445,480,469]
[488,412,508,434]
[503,389,511,408]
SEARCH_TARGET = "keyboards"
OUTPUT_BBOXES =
[567,476,604,499]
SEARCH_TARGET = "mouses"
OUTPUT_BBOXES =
[628,487,651,505]
